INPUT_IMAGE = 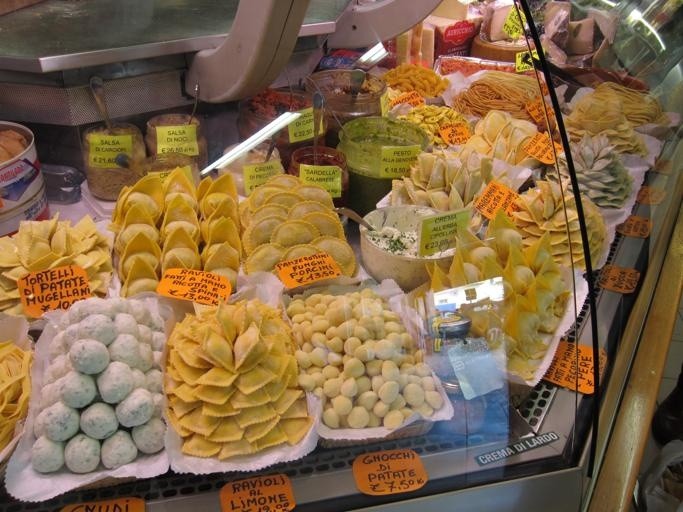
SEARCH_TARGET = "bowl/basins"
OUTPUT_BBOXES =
[358,203,455,290]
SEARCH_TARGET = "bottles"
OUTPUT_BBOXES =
[218,140,285,197]
[334,115,430,211]
[305,69,388,147]
[81,121,148,201]
[144,112,208,166]
[287,145,349,206]
[235,88,327,169]
[140,152,200,186]
